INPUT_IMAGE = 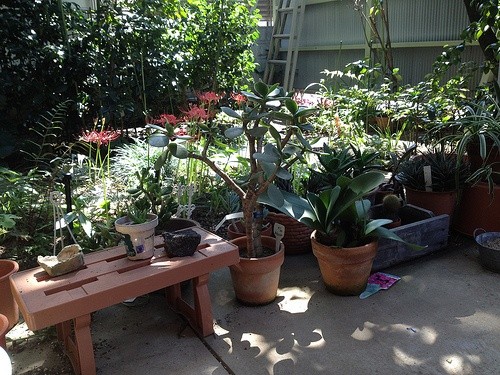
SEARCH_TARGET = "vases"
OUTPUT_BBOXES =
[0,261,21,328]
[1,315,10,351]
[473,228,500,273]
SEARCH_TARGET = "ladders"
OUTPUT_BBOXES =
[261,0,306,101]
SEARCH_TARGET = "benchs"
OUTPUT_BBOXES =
[9,226,240,374]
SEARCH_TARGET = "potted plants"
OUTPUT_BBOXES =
[257,140,428,298]
[428,91,500,237]
[113,198,160,261]
[126,151,201,235]
[395,144,472,229]
[383,194,402,227]
[218,194,272,237]
[144,80,330,307]
[373,89,398,134]
[262,174,314,254]
